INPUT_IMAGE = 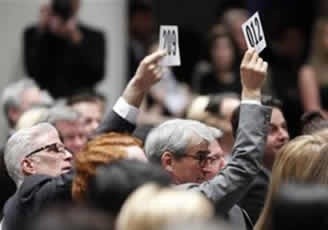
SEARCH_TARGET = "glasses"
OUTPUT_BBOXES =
[183,153,217,169]
[25,141,68,160]
[79,115,103,125]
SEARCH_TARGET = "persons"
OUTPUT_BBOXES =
[1,49,165,230]
[0,0,328,229]
[252,134,328,230]
[145,48,271,230]
